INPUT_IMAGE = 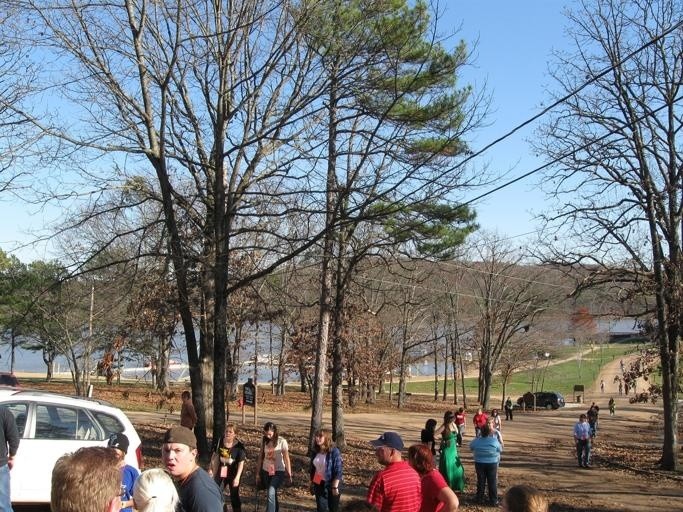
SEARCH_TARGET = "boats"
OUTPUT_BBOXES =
[104,354,190,373]
[235,352,291,368]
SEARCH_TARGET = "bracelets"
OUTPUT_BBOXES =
[9,456,15,461]
[332,487,338,489]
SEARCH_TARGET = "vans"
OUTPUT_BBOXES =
[1,384,148,512]
[516,390,565,410]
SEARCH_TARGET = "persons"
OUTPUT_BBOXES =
[408,445,459,512]
[214,424,246,511]
[609,397,615,415]
[587,407,598,437]
[0,406,19,511]
[345,500,376,512]
[420,406,503,505]
[368,433,422,512]
[310,429,343,512]
[505,397,513,420]
[163,427,225,512]
[132,469,178,512]
[573,414,593,468]
[108,433,138,512]
[181,392,198,430]
[600,380,605,393]
[256,422,292,512]
[505,485,549,512]
[591,402,600,426]
[52,446,122,512]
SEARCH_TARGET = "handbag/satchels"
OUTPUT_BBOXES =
[208,452,220,480]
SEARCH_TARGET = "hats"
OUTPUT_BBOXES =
[163,425,198,449]
[108,432,129,454]
[369,432,404,450]
